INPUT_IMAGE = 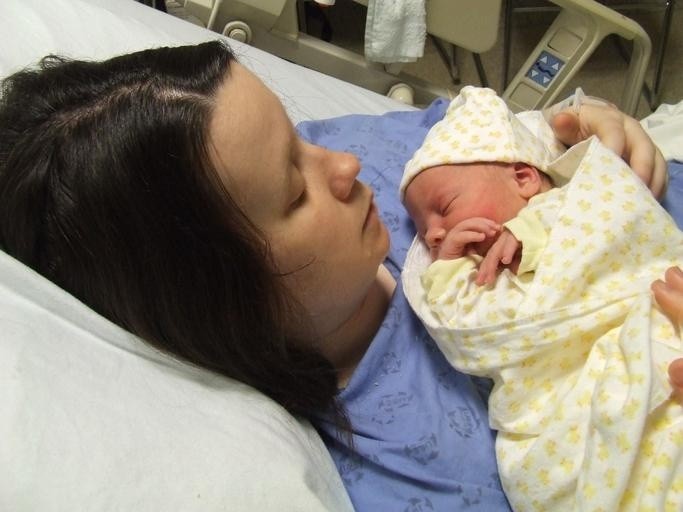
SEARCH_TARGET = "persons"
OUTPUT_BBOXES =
[400,87,682,511]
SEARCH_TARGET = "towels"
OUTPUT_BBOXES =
[365,0,427,65]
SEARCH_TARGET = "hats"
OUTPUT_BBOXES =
[400,85,552,198]
